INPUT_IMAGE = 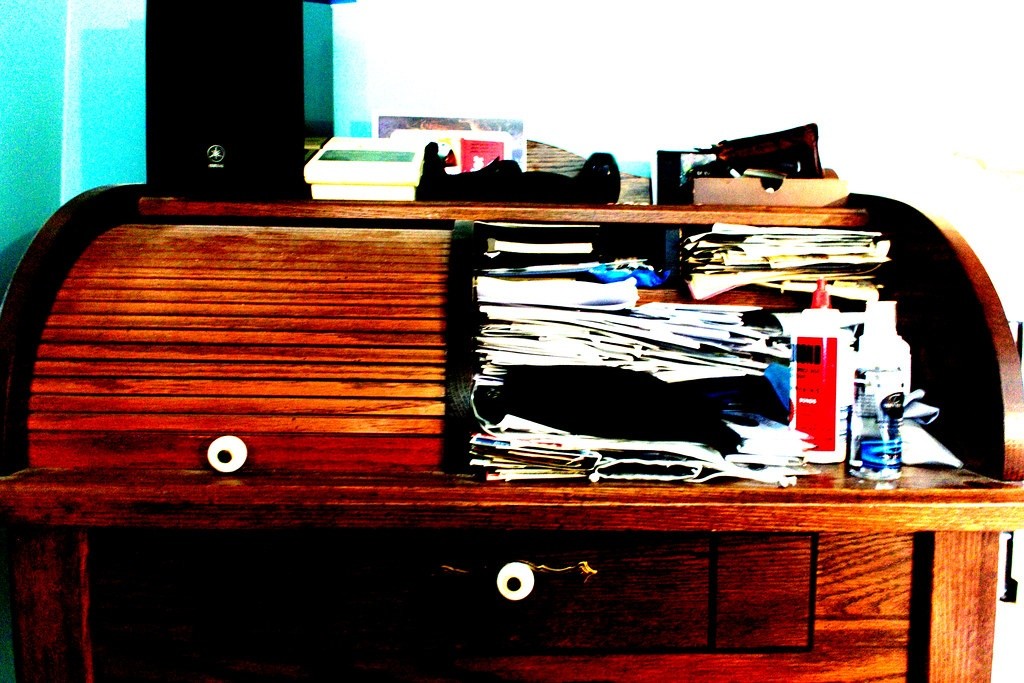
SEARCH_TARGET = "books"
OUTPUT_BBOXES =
[302,135,426,201]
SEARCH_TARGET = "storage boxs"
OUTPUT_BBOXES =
[693,179,851,207]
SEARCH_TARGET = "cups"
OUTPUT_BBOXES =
[849,396,902,481]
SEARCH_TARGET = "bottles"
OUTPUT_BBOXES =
[860,299,911,405]
[789,279,850,463]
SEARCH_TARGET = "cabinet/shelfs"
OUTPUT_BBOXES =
[0,193,1024,683]
